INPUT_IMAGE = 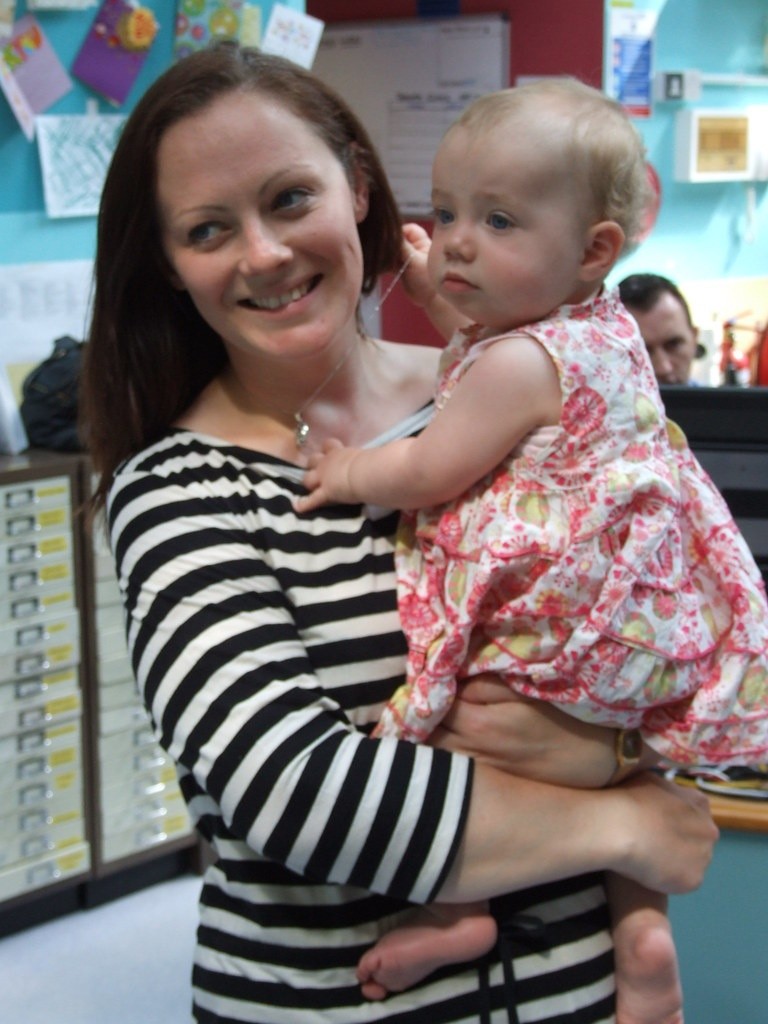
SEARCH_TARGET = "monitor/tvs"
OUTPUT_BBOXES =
[658,384,768,593]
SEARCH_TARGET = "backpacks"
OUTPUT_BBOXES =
[20,336,89,456]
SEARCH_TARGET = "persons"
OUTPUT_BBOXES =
[615,274,699,389]
[293,74,768,1024]
[70,41,721,1024]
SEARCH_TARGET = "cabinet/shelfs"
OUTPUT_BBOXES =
[1,451,95,941]
[68,450,201,912]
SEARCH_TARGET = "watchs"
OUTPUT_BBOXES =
[605,727,646,789]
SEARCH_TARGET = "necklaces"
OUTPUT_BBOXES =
[231,252,418,451]
